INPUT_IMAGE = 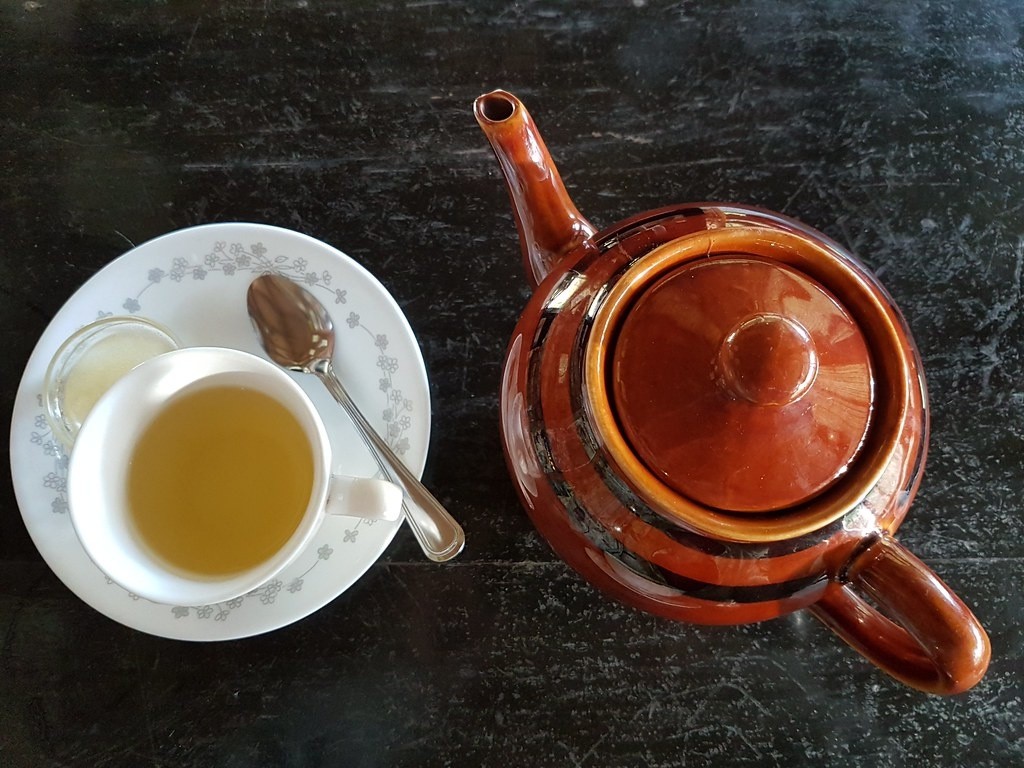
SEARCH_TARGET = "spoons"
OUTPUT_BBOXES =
[246,273,466,563]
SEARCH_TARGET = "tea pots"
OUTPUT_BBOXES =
[473,84,992,697]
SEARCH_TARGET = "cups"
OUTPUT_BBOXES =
[41,315,182,447]
[65,344,404,608]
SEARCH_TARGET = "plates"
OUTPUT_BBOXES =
[7,222,432,642]
